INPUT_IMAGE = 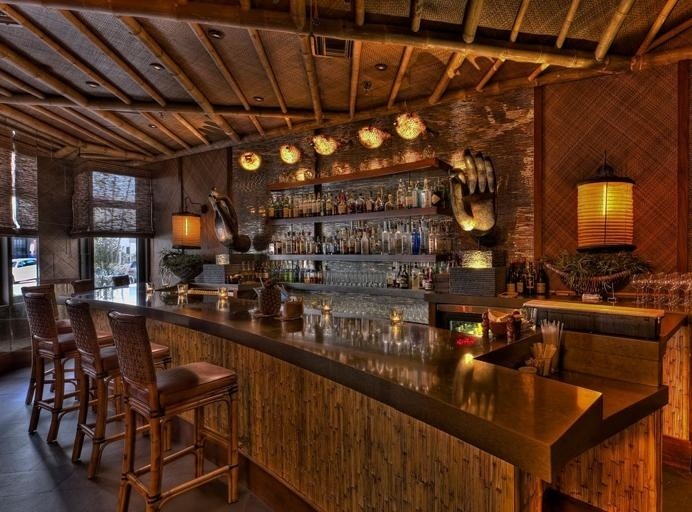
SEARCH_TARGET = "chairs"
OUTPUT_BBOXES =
[22,293,121,442]
[113,276,129,287]
[21,284,78,405]
[65,300,172,479]
[107,310,239,512]
[72,278,93,293]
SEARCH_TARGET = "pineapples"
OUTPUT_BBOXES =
[258,275,280,314]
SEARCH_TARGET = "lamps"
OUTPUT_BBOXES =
[172,196,208,250]
[575,149,637,253]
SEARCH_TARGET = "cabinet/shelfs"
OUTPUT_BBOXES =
[260,158,452,300]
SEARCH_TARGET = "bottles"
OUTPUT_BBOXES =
[270,216,438,254]
[526,263,534,293]
[321,347,437,393]
[268,177,433,218]
[506,268,515,292]
[239,260,456,290]
[516,268,524,291]
[511,311,521,334]
[537,262,547,295]
[303,313,449,359]
[506,316,514,337]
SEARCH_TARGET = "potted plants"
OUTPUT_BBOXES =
[542,254,662,297]
[159,248,203,284]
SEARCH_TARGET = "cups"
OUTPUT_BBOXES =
[253,287,280,315]
[280,298,304,316]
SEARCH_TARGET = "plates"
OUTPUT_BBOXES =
[500,292,517,298]
[249,309,281,317]
[488,309,508,322]
[275,316,302,320]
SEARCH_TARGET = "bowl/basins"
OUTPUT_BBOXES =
[489,320,507,336]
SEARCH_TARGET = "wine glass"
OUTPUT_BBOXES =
[631,271,692,313]
